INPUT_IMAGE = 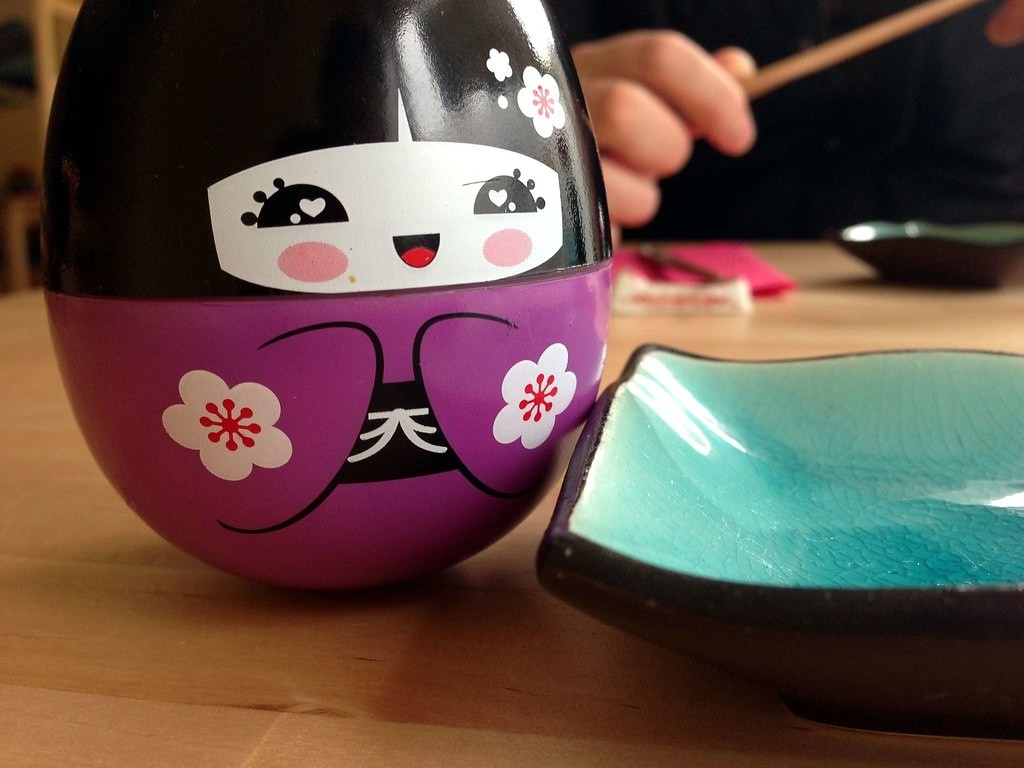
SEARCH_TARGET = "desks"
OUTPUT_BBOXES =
[0,241,1024,768]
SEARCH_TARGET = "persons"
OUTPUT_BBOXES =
[547,1,1024,244]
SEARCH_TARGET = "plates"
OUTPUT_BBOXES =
[832,220,1023,287]
[537,344,1024,739]
